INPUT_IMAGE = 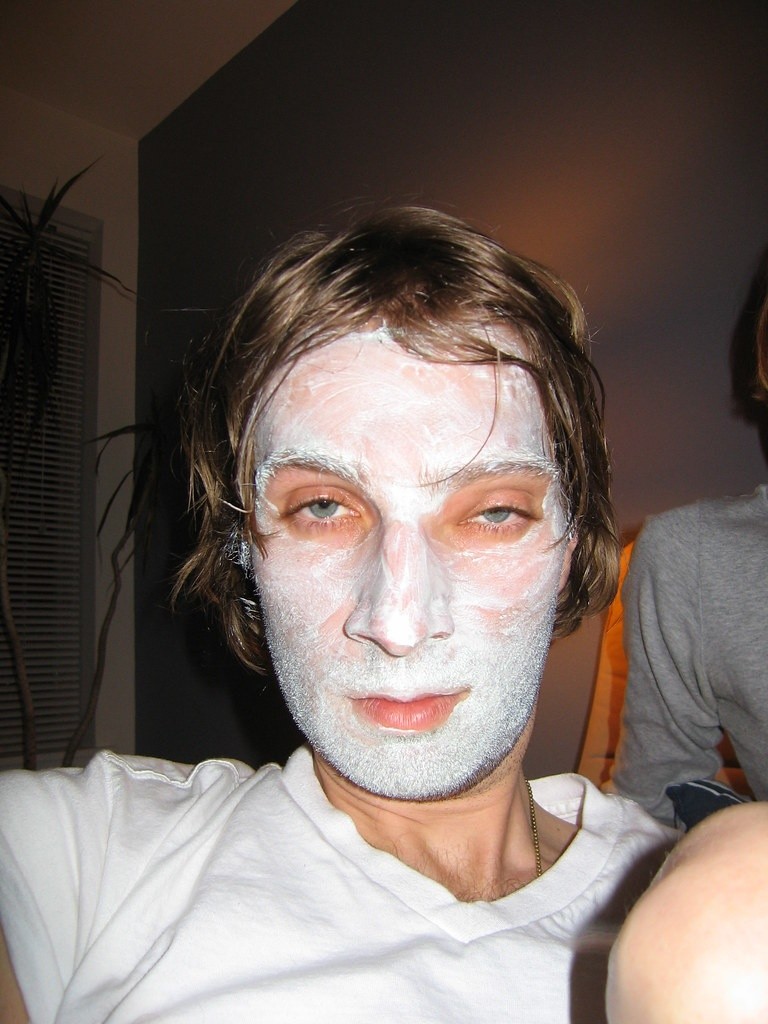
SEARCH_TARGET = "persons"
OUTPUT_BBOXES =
[0,208,768,1024]
[602,246,768,835]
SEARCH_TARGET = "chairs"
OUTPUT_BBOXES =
[576,540,755,803]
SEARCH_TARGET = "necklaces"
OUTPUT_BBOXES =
[524,778,542,877]
